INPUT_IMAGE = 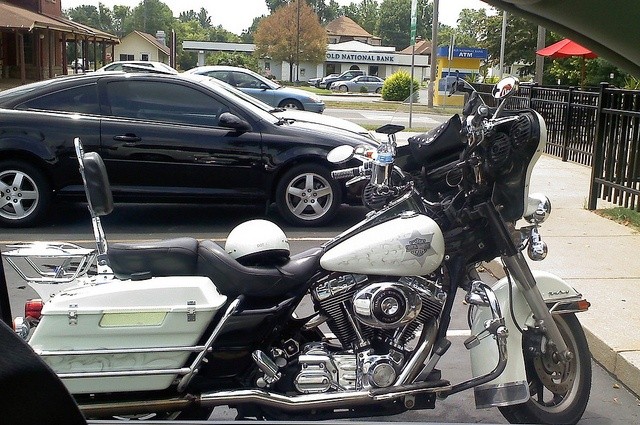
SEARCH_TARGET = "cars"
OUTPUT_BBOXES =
[93,60,179,76]
[329,75,386,94]
[0,73,393,228]
[181,65,325,115]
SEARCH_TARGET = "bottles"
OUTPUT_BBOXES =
[371,135,390,188]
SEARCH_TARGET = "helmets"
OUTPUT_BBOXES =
[225,219,290,264]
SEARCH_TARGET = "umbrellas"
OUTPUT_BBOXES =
[536,38,600,84]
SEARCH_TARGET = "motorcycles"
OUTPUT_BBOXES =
[0,76,592,423]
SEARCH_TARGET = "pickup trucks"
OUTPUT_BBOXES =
[324,70,366,91]
